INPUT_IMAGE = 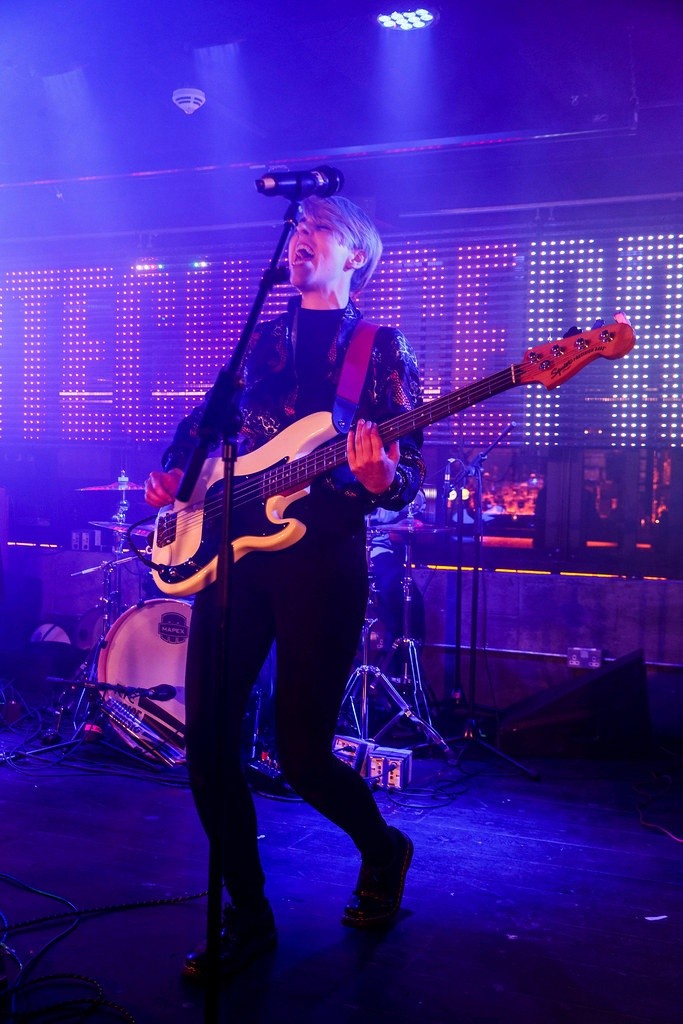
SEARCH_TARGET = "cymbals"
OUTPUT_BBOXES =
[88,517,156,541]
[73,477,148,499]
[362,513,455,540]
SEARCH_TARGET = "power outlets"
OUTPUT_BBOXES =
[567,649,602,669]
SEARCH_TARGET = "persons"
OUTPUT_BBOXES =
[144,196,426,980]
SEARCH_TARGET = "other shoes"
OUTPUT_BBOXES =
[178,902,277,983]
[341,825,415,925]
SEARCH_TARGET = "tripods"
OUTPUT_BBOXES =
[0,678,172,772]
[342,422,543,784]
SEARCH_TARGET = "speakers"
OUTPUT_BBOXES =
[482,649,658,757]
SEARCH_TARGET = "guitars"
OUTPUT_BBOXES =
[148,321,636,594]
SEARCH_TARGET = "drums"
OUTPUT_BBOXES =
[89,587,214,770]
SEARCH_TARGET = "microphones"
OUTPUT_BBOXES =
[443,462,452,498]
[255,164,345,197]
[136,683,177,702]
